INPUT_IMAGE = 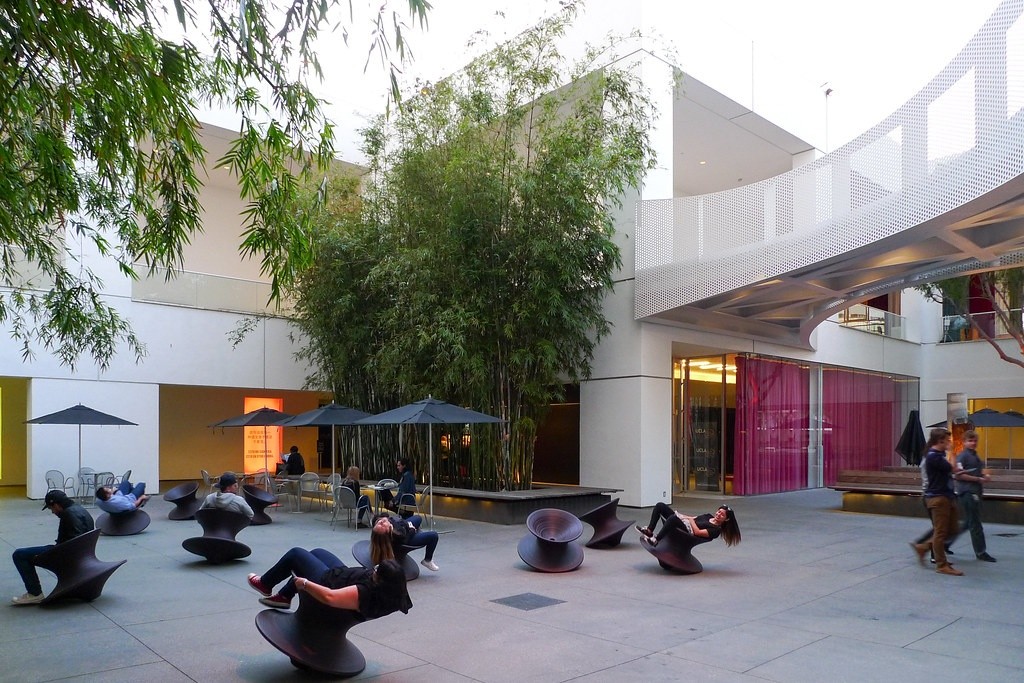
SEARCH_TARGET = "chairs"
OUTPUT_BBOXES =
[77,467,95,498]
[106,470,131,490]
[92,472,114,504]
[201,467,430,532]
[43,470,76,504]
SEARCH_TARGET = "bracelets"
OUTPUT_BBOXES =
[303,581,306,590]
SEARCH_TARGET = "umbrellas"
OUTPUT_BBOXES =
[22,402,139,505]
[1002,408,1024,471]
[207,406,292,491]
[894,407,926,467]
[926,404,1024,469]
[267,400,375,511]
[353,394,509,531]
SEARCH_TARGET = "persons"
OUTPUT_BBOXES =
[370,512,439,571]
[340,466,375,529]
[95,480,150,514]
[635,502,741,547]
[945,430,997,562]
[198,471,254,520]
[283,446,305,475]
[909,429,965,576]
[247,546,412,616]
[9,490,94,604]
[391,458,416,519]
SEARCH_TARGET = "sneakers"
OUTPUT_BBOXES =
[259,593,290,609]
[247,572,272,596]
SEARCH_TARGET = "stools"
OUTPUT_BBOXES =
[517,508,584,572]
[31,529,127,602]
[241,484,278,526]
[256,568,380,676]
[95,499,151,535]
[640,514,713,574]
[181,508,252,563]
[353,540,425,582]
[163,482,205,520]
[580,498,635,549]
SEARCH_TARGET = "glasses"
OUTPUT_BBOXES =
[722,503,733,512]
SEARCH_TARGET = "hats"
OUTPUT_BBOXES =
[214,473,238,488]
[42,490,66,511]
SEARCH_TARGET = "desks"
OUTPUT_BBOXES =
[79,472,102,508]
[367,485,395,518]
[276,478,305,513]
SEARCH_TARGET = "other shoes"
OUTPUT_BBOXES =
[368,511,374,518]
[141,495,151,508]
[12,591,45,603]
[421,559,439,571]
[635,526,654,539]
[911,543,927,565]
[930,557,954,565]
[402,510,414,520]
[936,563,963,576]
[945,547,954,555]
[976,552,996,563]
[644,535,659,548]
[354,520,371,529]
